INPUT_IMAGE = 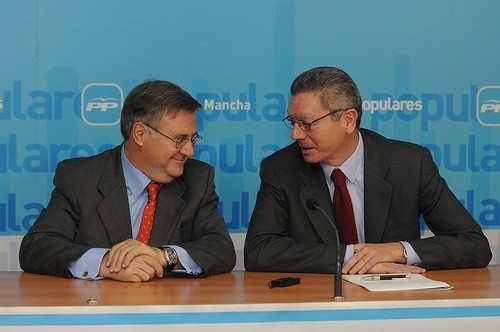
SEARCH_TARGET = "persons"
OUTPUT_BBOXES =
[244,66,493,276]
[20,80,237,282]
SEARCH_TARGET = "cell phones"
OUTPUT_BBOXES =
[270,277,301,286]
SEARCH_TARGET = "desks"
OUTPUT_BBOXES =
[0,264,500,332]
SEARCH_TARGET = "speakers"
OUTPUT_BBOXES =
[304,198,344,299]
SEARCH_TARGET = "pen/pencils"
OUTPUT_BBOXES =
[362,275,411,280]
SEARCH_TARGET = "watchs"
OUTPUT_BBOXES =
[158,246,178,272]
[403,249,407,258]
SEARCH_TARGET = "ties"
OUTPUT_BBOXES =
[136,184,161,246]
[330,168,359,245]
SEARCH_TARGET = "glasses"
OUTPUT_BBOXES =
[281,109,344,131]
[141,122,203,149]
